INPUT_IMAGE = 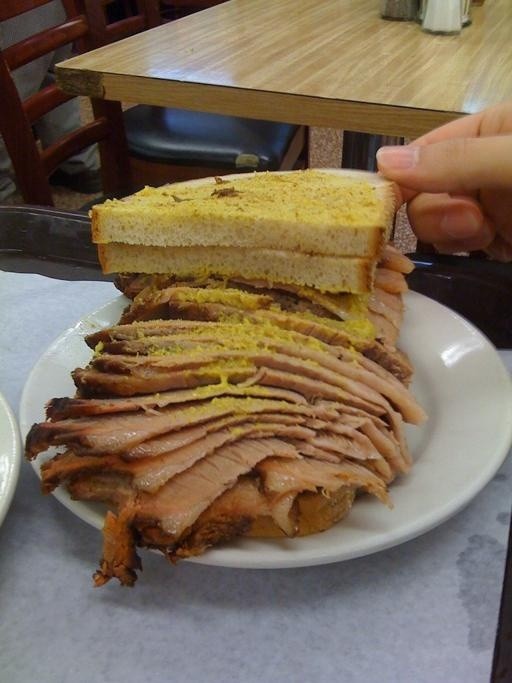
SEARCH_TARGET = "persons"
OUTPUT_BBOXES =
[371,96,512,264]
[1,0,104,202]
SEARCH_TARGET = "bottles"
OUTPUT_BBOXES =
[377,2,472,35]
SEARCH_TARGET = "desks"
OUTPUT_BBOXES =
[56,0,510,237]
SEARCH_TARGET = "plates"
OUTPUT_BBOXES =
[0,390,21,532]
[22,288,510,571]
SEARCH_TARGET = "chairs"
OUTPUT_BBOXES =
[1,0,293,220]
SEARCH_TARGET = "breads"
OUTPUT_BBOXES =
[90,169,397,296]
